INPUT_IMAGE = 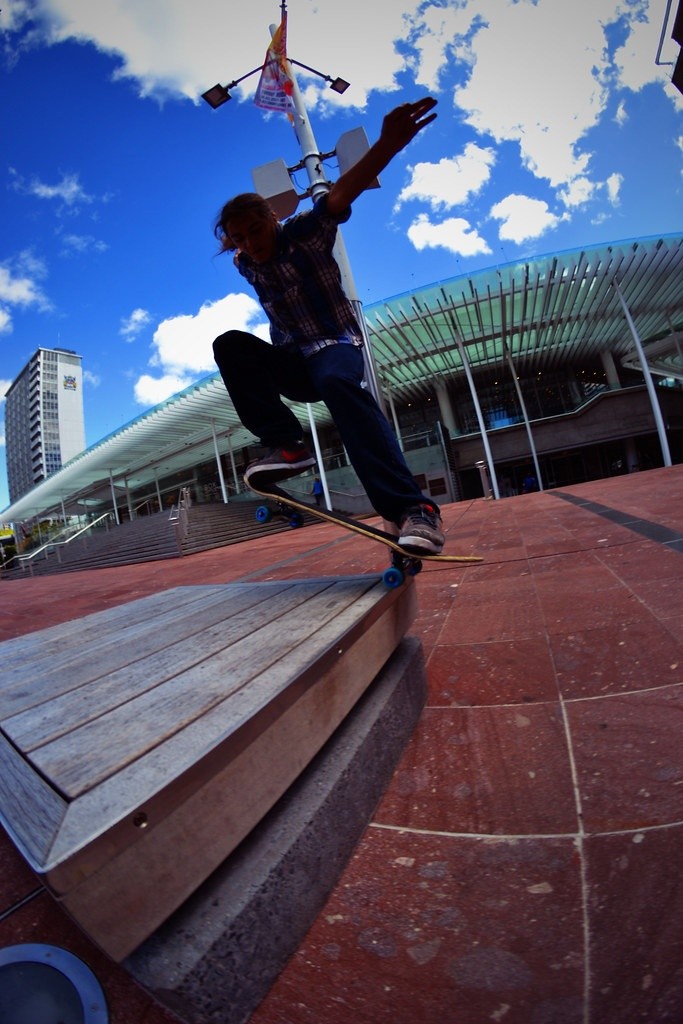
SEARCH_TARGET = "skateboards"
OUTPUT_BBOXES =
[241,474,486,591]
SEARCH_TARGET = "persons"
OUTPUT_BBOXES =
[310,478,323,506]
[213,97,445,556]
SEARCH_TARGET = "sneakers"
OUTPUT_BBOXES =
[397,500,445,555]
[246,439,317,484]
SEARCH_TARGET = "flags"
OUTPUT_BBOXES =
[255,12,301,149]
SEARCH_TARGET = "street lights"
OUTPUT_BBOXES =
[200,24,399,562]
